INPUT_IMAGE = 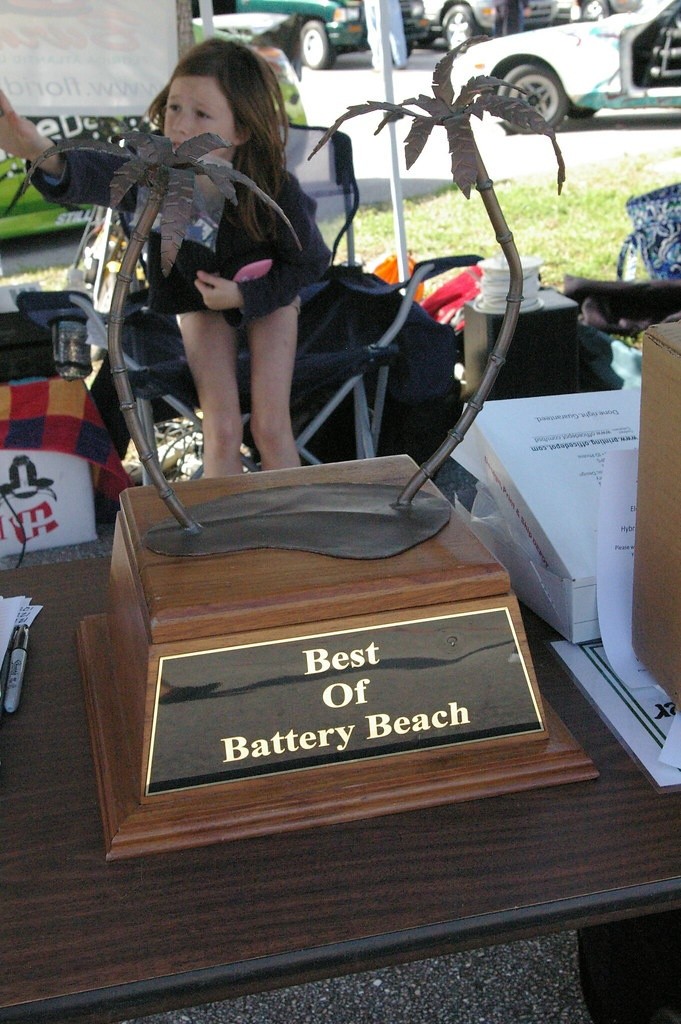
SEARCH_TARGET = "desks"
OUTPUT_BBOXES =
[0,562,681,1024]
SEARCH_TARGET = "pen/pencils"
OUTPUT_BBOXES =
[4,624,30,714]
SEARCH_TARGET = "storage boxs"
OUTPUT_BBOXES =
[459,319,679,717]
[0,375,98,557]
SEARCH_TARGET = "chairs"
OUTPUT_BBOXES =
[131,123,485,486]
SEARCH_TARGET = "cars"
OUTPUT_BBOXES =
[189,0,613,72]
[464,0,681,133]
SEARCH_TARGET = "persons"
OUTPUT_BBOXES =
[0,38,332,479]
